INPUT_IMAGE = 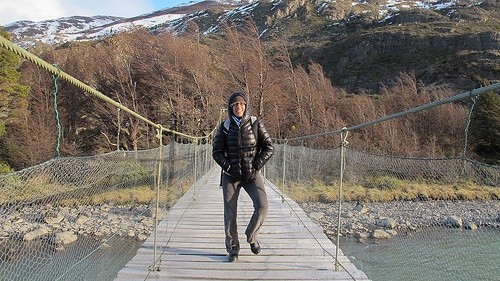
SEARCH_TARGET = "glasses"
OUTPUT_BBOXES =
[234,103,246,106]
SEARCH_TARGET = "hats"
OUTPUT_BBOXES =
[230,96,246,106]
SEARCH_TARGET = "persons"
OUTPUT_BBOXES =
[211,92,275,262]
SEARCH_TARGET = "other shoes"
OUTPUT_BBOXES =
[250,240,260,254]
[228,253,238,262]
